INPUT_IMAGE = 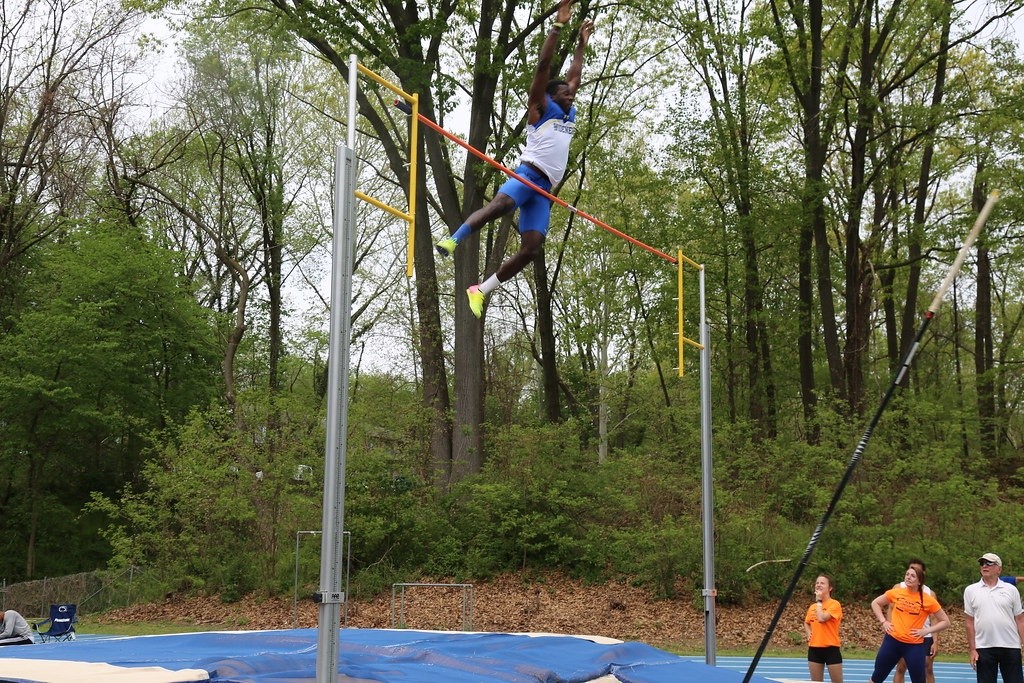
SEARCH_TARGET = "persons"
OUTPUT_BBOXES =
[437,0,595,320]
[805,552,1024,683]
[0,609,35,646]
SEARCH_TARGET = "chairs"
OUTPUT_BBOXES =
[31,604,77,643]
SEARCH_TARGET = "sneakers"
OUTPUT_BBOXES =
[436,238,459,257]
[466,284,485,318]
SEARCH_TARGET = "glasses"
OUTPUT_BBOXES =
[980,561,997,566]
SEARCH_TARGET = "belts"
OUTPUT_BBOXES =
[521,161,549,181]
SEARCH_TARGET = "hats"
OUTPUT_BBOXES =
[978,553,1002,567]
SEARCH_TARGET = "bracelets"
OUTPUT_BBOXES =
[551,23,564,34]
[881,619,886,625]
[817,600,822,606]
